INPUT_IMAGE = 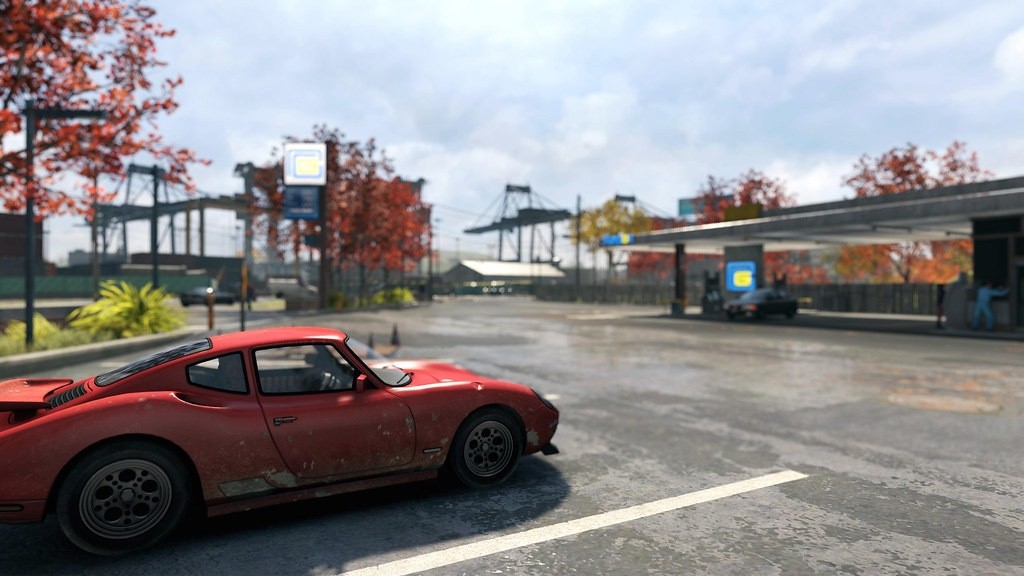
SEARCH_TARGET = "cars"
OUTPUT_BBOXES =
[722,287,799,322]
[267,277,319,298]
[179,286,235,307]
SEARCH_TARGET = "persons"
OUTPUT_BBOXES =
[971,281,1012,333]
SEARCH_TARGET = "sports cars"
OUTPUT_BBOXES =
[0,325,561,560]
